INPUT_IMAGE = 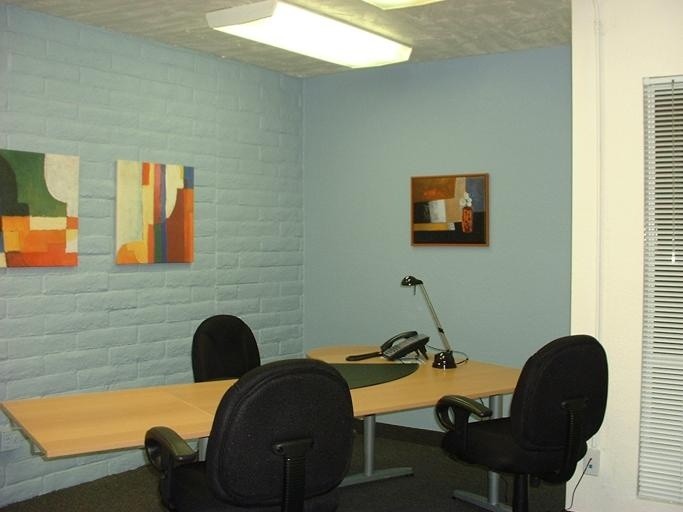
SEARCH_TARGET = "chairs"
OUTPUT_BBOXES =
[145,358,355,512]
[192,315,262,384]
[436,334,609,512]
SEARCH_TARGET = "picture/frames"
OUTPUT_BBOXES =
[410,174,490,247]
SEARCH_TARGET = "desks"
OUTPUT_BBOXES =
[0,344,523,512]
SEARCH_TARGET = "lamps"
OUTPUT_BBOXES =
[399,273,469,370]
[204,1,413,70]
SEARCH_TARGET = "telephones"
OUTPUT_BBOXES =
[381,331,429,361]
